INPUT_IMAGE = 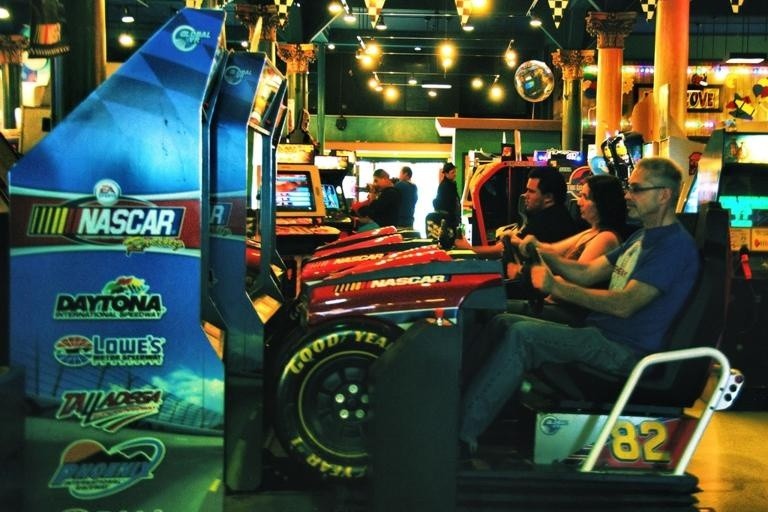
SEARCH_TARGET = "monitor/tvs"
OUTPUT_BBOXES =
[681,173,718,214]
[275,171,316,212]
[321,183,340,209]
[718,194,768,229]
[247,130,262,210]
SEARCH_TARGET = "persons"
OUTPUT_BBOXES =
[433,162,462,240]
[352,166,417,230]
[458,158,700,468]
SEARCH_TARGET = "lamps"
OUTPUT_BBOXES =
[121,6,134,25]
[375,16,385,30]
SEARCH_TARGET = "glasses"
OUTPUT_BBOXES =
[622,182,667,193]
